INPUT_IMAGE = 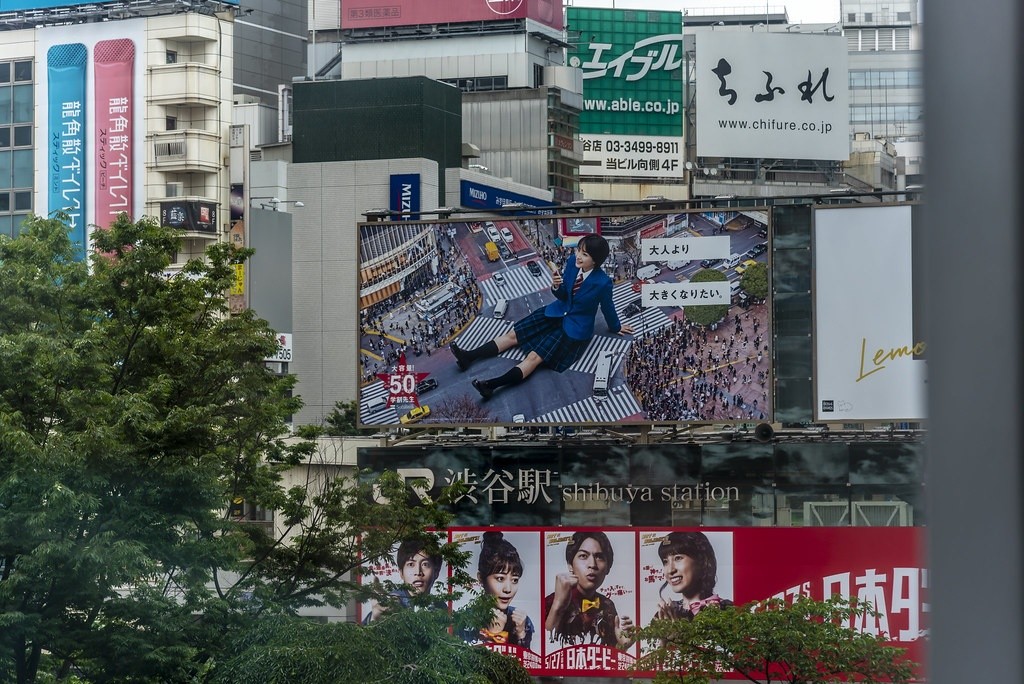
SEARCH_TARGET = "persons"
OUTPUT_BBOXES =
[362,537,447,626]
[651,530,734,650]
[544,531,636,651]
[359,224,768,419]
[450,530,535,651]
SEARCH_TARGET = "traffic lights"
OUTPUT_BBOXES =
[230,494,245,519]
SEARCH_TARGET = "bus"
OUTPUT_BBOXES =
[592,349,613,403]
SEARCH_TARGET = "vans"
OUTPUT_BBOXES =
[666,258,691,271]
[723,252,741,269]
[483,242,499,261]
[636,264,661,282]
[493,298,508,319]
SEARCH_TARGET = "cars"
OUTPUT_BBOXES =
[621,299,647,318]
[758,226,767,239]
[632,278,655,292]
[498,244,511,259]
[400,405,431,425]
[512,413,525,425]
[700,257,722,269]
[486,226,502,243]
[501,227,514,244]
[527,261,542,277]
[471,222,480,230]
[734,259,758,275]
[492,271,506,286]
[746,243,768,259]
[370,431,391,439]
[413,377,438,396]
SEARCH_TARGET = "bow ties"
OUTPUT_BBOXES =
[582,597,600,612]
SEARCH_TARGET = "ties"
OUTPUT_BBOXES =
[571,271,583,301]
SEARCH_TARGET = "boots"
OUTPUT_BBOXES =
[471,366,523,402]
[450,340,501,372]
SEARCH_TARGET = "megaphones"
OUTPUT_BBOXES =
[754,423,774,443]
